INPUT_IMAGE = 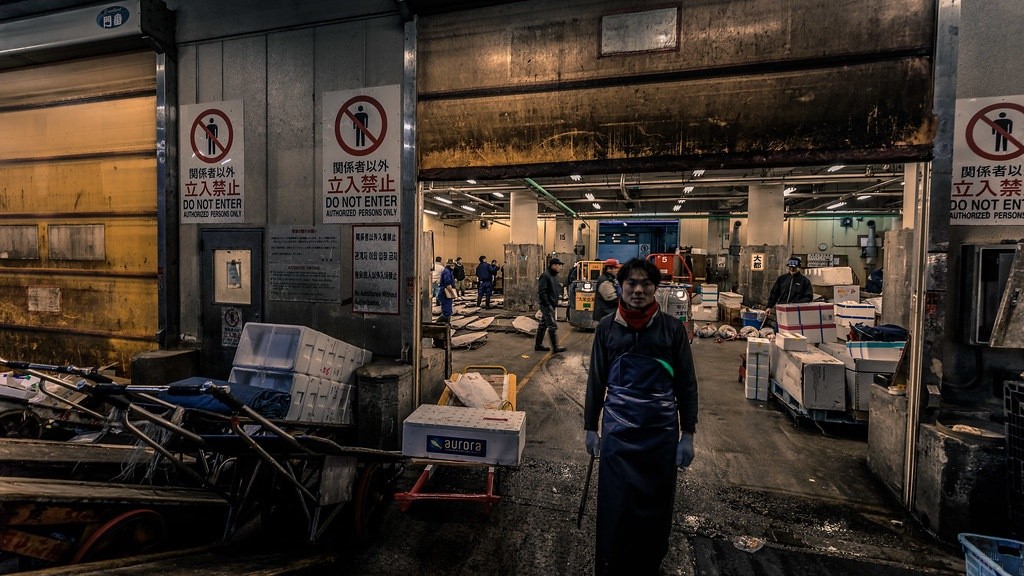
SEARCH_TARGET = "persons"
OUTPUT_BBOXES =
[476,256,492,309]
[491,259,504,293]
[436,259,456,323]
[566,262,581,322]
[446,257,465,297]
[593,259,621,327]
[432,256,445,306]
[766,258,814,334]
[867,267,883,294]
[583,257,698,576]
[535,258,567,354]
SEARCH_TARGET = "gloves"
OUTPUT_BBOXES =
[585,431,600,457]
[675,434,695,469]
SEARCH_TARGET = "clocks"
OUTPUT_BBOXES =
[818,242,827,251]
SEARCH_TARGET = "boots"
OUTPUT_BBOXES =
[550,331,567,353]
[535,332,550,351]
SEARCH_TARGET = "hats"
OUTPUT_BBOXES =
[605,259,618,266]
[550,259,564,265]
[786,257,801,268]
[457,257,462,261]
[446,259,455,265]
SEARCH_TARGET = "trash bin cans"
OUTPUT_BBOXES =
[741,309,766,330]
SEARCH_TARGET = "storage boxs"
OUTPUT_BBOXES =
[226,321,373,425]
[690,283,719,321]
[719,305,743,326]
[718,291,743,309]
[739,265,906,412]
[400,403,526,466]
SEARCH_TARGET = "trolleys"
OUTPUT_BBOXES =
[0,354,403,558]
[394,365,517,513]
[646,253,695,346]
